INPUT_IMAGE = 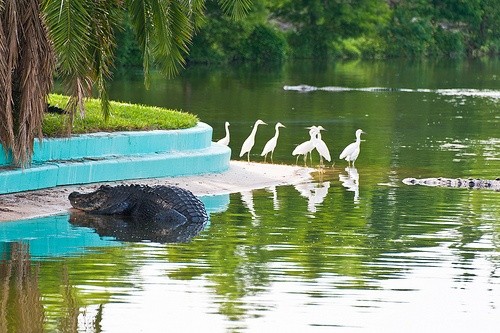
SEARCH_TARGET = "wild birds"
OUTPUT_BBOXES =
[216,119,365,167]
